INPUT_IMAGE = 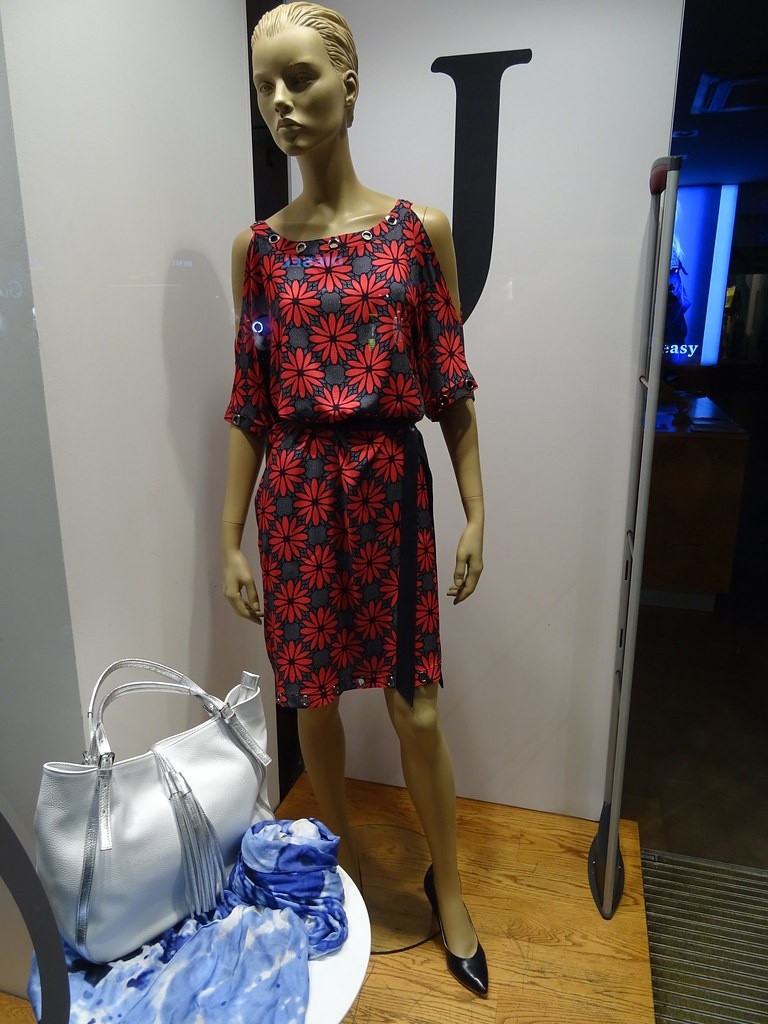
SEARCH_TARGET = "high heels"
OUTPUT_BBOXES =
[423,863,489,996]
[356,854,364,896]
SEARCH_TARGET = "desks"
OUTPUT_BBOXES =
[29,865,371,1024]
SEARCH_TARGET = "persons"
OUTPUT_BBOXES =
[222,3,484,998]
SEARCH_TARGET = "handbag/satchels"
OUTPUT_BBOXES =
[34,659,276,964]
